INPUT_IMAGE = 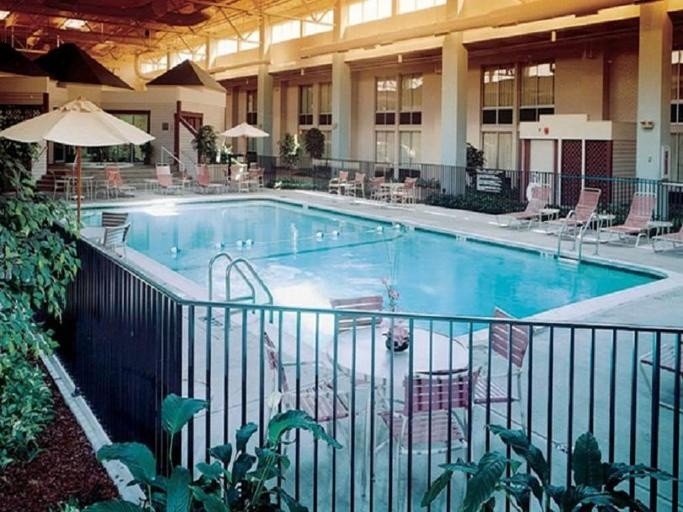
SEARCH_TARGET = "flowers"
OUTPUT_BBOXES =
[377,277,416,348]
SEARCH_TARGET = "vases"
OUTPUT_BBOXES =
[385,330,409,355]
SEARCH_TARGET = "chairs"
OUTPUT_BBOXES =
[629,342,681,416]
[257,329,357,456]
[46,158,266,199]
[482,182,682,253]
[99,209,130,226]
[328,291,386,407]
[326,169,419,207]
[458,304,536,439]
[103,221,131,258]
[373,364,483,509]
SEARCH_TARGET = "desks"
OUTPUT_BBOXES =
[77,224,105,239]
[321,322,473,499]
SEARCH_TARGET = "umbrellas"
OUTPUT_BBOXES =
[219,122,270,164]
[0,94,156,229]
